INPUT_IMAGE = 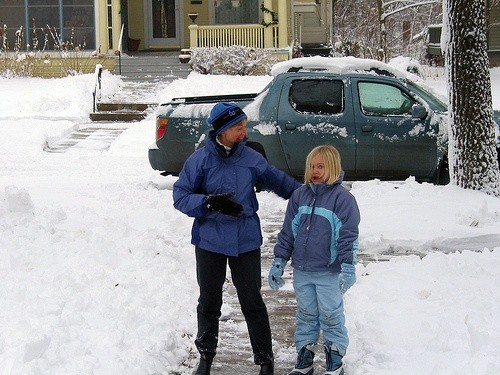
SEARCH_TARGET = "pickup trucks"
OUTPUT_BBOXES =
[148,66,500,185]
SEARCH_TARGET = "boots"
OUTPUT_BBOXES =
[286,344,316,375]
[322,342,345,375]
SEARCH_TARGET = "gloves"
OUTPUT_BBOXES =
[338,262,356,294]
[203,191,243,217]
[268,258,287,290]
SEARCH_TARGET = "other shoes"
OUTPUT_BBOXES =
[193,358,212,375]
[254,354,275,375]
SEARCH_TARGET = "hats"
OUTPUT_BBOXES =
[207,102,247,137]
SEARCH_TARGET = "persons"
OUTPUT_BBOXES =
[269,146,360,375]
[173,102,303,375]
